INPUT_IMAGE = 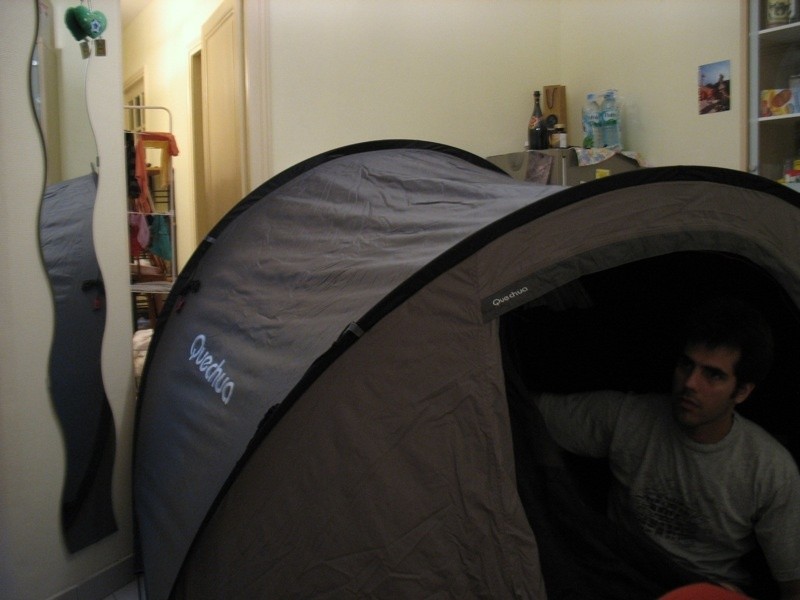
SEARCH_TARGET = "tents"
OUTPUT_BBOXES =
[129,138,800,599]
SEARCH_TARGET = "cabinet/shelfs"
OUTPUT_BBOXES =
[123,104,176,359]
[749,0,800,192]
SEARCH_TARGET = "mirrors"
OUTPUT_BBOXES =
[30,0,120,554]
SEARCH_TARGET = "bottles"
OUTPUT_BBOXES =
[582,89,621,149]
[527,90,550,149]
[547,124,568,148]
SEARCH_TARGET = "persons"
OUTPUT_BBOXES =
[522,311,800,599]
